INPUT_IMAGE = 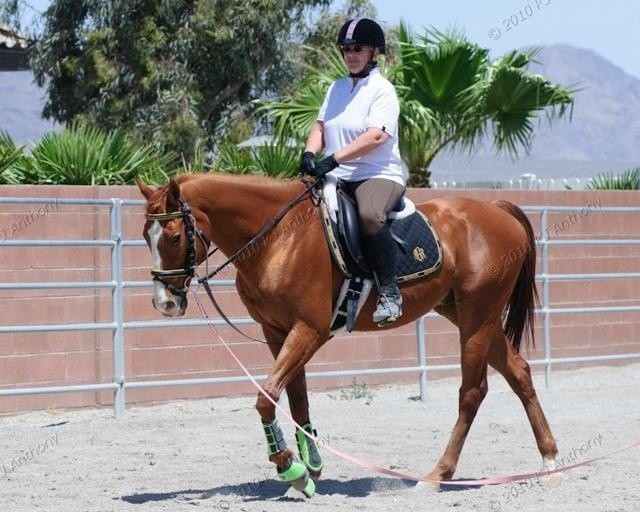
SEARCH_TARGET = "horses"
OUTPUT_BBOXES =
[134,172,559,501]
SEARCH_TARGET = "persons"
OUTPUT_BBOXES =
[298,17,410,323]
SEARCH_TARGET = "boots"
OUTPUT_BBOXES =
[359,232,403,323]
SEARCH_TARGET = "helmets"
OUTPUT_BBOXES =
[336,19,385,54]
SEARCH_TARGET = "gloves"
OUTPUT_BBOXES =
[299,151,339,181]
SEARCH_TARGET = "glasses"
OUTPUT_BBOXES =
[342,45,362,53]
